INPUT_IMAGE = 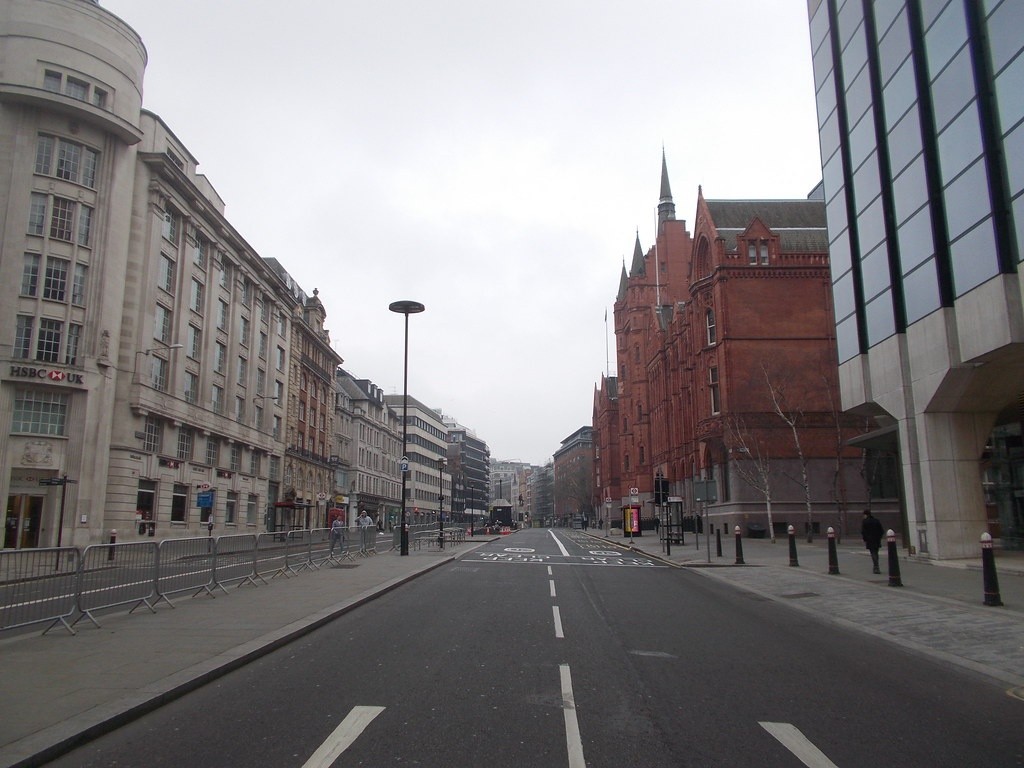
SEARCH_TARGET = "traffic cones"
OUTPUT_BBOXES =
[500,526,511,535]
[467,527,471,536]
[486,527,490,535]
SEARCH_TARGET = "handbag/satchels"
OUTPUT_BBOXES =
[332,520,344,535]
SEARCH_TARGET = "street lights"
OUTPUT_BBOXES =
[470,480,477,536]
[389,300,425,556]
[436,458,447,548]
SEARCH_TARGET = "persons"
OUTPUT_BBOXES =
[354,510,374,525]
[329,514,346,554]
[860,508,884,574]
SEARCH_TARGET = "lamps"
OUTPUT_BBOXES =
[253,396,279,402]
[146,344,183,355]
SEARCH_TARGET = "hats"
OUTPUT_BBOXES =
[360,511,366,513]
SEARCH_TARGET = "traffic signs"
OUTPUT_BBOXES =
[39,477,79,486]
